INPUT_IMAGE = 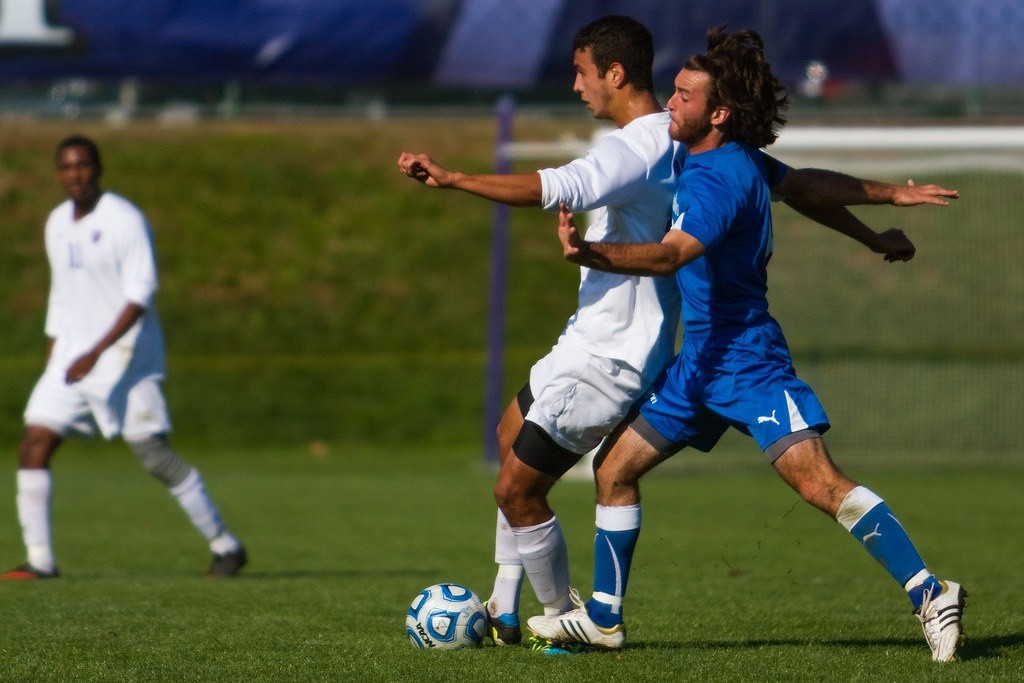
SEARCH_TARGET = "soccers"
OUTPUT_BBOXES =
[404,581,489,651]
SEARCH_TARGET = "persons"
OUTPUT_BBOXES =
[527,23,962,663]
[397,16,916,654]
[0,134,248,580]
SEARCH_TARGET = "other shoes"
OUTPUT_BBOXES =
[205,544,249,578]
[0,564,59,580]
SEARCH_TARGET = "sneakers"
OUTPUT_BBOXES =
[527,624,583,655]
[912,579,969,664]
[525,585,627,650]
[480,600,522,646]
